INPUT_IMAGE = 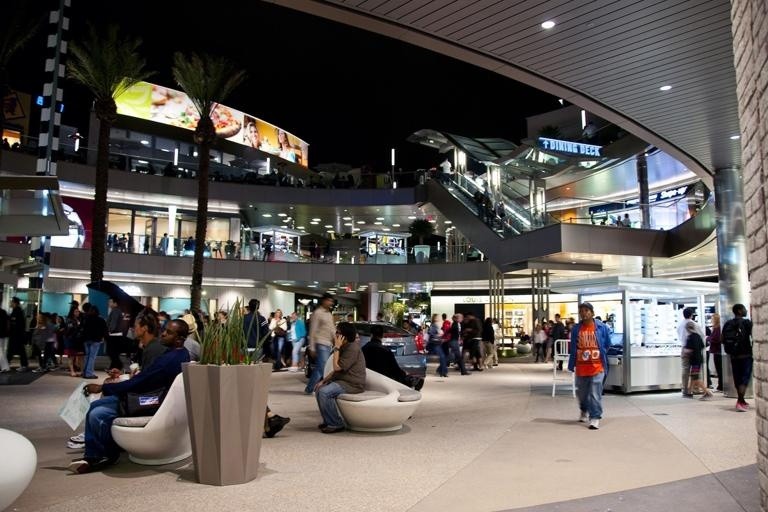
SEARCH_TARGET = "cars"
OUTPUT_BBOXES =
[303,321,427,392]
[404,312,426,326]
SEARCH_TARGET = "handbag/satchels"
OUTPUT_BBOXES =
[119,386,168,417]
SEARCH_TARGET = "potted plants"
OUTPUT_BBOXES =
[407,217,436,265]
[179,295,287,488]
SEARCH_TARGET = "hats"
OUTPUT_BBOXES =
[579,302,592,311]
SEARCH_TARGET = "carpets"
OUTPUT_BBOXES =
[0,365,49,386]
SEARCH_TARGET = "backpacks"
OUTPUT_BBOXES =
[724,319,746,355]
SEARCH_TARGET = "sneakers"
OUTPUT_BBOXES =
[700,391,714,401]
[736,401,749,413]
[319,423,344,434]
[265,414,290,438]
[580,410,589,423]
[16,366,59,373]
[66,432,120,473]
[588,418,600,429]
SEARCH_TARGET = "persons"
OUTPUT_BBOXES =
[263,238,273,261]
[147,161,179,178]
[206,238,222,258]
[600,214,629,227]
[272,128,296,161]
[178,299,306,372]
[315,321,366,433]
[519,331,532,344]
[720,301,754,412]
[305,294,337,395]
[439,158,452,185]
[473,190,506,226]
[160,233,169,256]
[268,170,354,190]
[1,297,170,380]
[263,406,290,439]
[534,314,575,369]
[69,318,189,474]
[404,312,500,377]
[678,308,723,401]
[143,235,150,254]
[106,233,131,253]
[242,122,261,148]
[568,302,613,430]
[360,327,424,390]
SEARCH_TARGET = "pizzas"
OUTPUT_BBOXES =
[150,86,241,138]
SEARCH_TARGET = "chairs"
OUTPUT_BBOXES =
[550,339,579,399]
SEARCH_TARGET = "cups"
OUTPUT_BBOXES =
[129,363,141,376]
